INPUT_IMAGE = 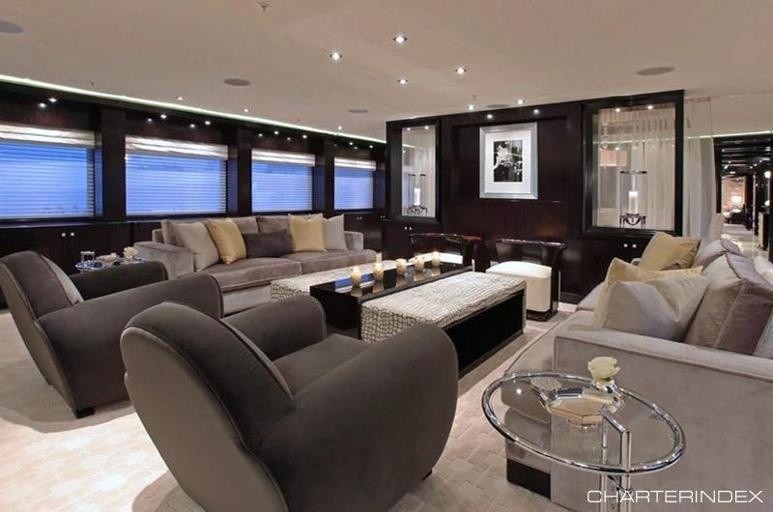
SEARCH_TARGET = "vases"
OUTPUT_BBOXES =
[592,382,618,393]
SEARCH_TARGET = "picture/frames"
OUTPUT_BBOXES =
[479,122,539,200]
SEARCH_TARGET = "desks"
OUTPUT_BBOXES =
[482,372,687,512]
[75,258,146,275]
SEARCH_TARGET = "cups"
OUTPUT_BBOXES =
[349,265,362,288]
[373,263,384,281]
[413,255,424,272]
[395,258,407,276]
[431,252,440,268]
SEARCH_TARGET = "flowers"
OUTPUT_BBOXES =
[588,357,621,380]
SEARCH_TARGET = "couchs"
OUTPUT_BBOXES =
[501,231,773,512]
[134,214,378,315]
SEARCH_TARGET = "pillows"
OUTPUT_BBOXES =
[324,213,348,250]
[288,213,325,252]
[242,229,292,258]
[602,232,773,357]
[203,218,247,265]
[171,221,219,275]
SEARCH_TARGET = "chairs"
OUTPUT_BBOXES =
[0,250,224,419]
[120,292,461,512]
[488,237,568,322]
[408,233,484,274]
